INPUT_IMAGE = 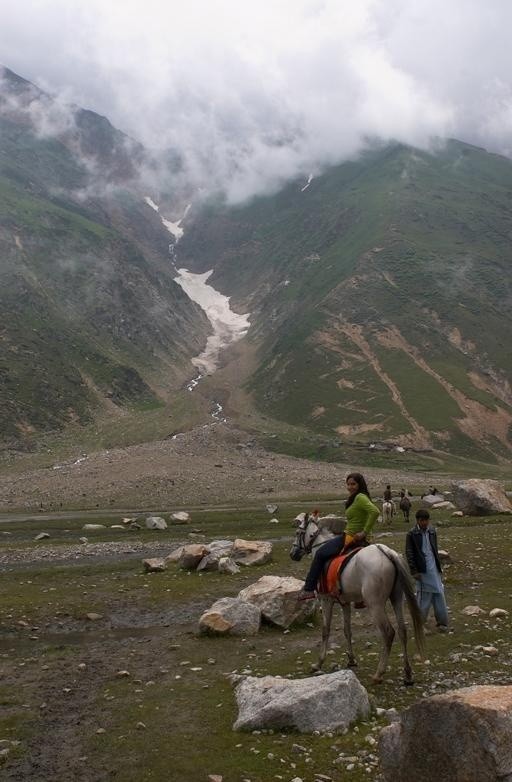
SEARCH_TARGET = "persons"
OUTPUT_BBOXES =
[405,509,450,630]
[297,472,380,601]
[383,485,439,506]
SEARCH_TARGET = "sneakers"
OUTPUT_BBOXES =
[422,626,432,635]
[438,624,450,634]
[298,592,317,602]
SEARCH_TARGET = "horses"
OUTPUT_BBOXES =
[398,491,412,522]
[289,511,429,687]
[421,488,440,499]
[382,500,392,523]
